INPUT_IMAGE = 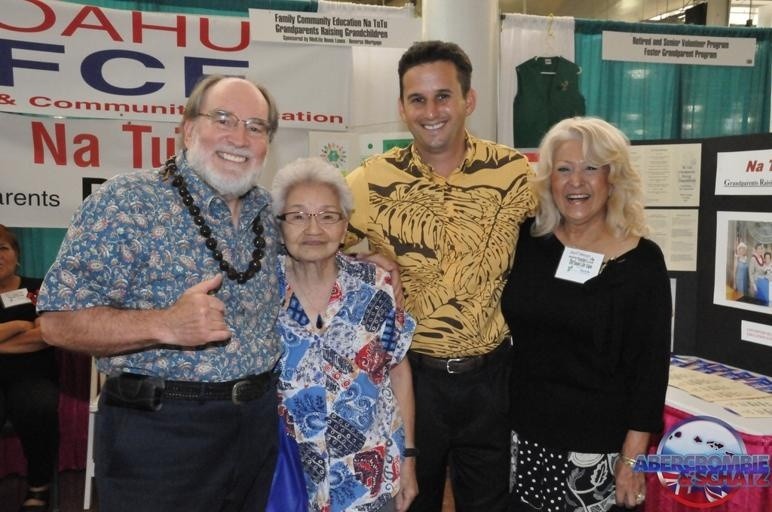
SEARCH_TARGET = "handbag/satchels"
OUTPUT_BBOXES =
[264,432,308,512]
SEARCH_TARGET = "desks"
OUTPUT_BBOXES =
[642,356,772,511]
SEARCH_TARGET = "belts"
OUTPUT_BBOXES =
[410,333,511,374]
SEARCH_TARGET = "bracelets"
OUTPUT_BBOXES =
[401,447,421,459]
[617,453,645,469]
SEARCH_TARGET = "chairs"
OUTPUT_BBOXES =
[0,328,108,510]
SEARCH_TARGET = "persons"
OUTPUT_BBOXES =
[334,36,544,512]
[0,221,65,512]
[36,70,409,510]
[730,241,772,305]
[499,114,675,511]
[260,159,420,511]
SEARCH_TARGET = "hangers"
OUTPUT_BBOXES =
[516,12,583,75]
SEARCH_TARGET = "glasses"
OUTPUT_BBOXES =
[277,210,344,224]
[198,110,272,138]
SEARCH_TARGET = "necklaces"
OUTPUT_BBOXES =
[295,273,333,330]
[166,157,269,284]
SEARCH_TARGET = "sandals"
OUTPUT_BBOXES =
[117,369,273,406]
[24,486,50,511]
